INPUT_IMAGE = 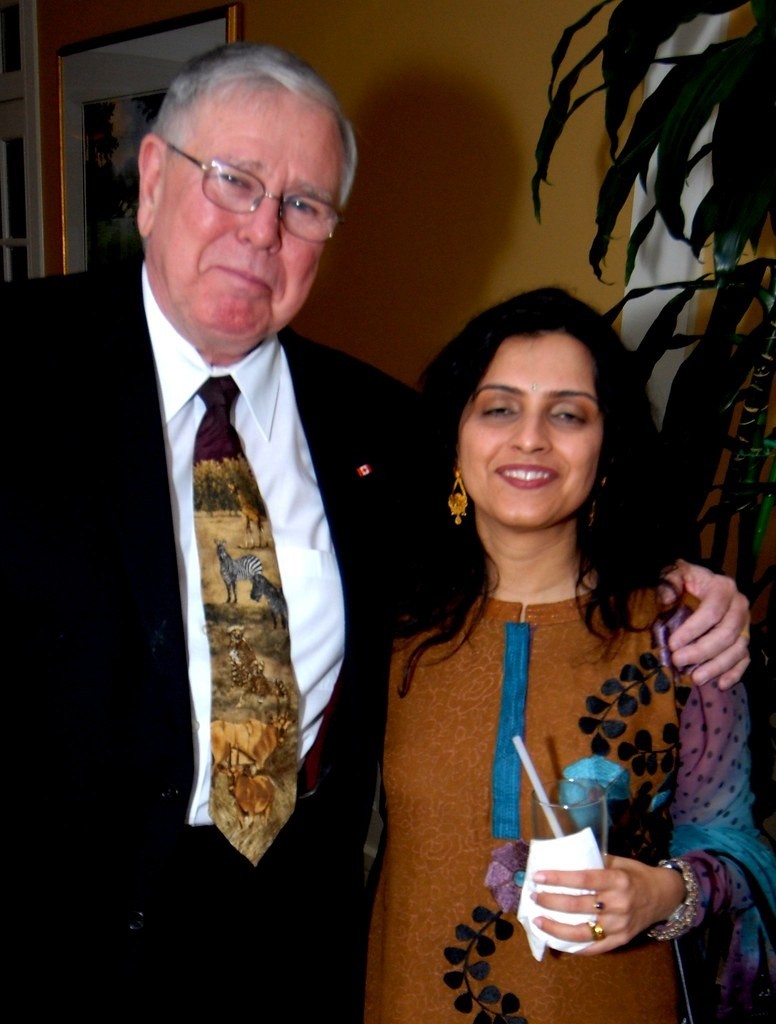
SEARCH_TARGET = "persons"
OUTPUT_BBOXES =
[0,42,751,1024]
[359,286,776,1024]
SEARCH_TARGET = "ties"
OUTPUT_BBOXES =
[193,376,299,868]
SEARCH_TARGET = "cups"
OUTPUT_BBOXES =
[530,777,608,954]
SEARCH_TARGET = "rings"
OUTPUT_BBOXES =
[586,921,605,942]
[592,892,605,918]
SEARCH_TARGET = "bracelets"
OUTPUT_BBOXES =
[646,859,699,942]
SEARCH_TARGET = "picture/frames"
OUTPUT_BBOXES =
[57,0,245,276]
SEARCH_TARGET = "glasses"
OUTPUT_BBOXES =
[166,141,344,243]
